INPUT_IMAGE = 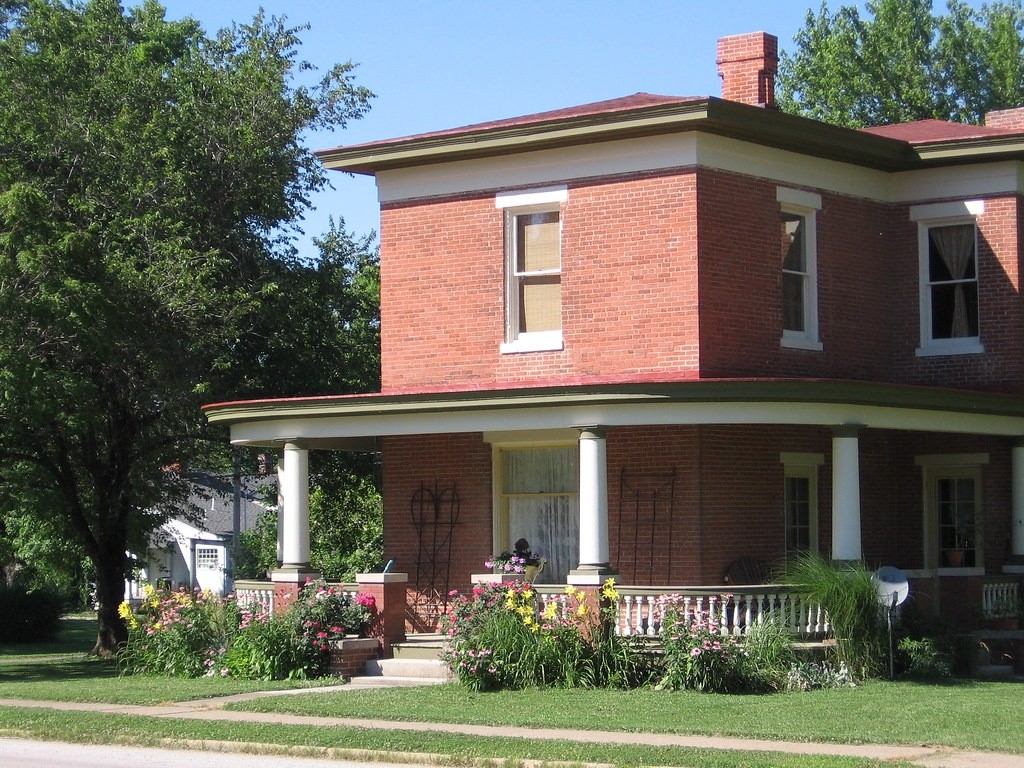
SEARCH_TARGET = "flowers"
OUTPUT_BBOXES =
[485,538,540,572]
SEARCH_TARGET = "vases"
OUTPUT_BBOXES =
[945,551,964,567]
[510,565,538,583]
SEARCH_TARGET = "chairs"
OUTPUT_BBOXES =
[722,559,786,631]
